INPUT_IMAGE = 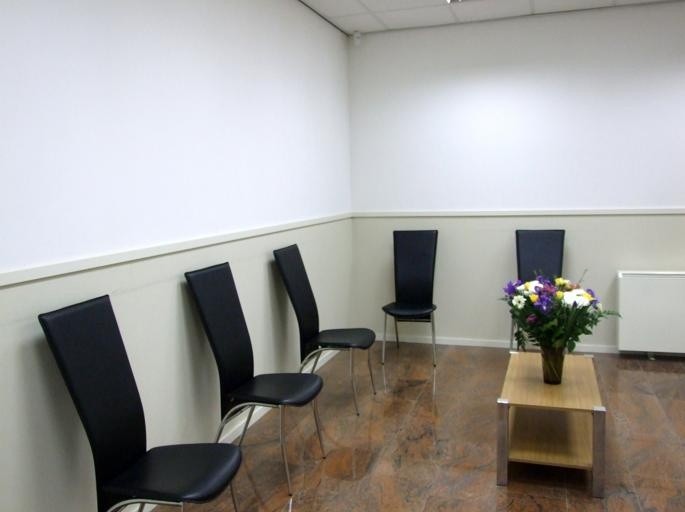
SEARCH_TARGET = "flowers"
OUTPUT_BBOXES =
[501,267,623,379]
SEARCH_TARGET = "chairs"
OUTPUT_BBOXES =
[509,229,566,348]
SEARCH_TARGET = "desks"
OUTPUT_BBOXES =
[495,350,609,499]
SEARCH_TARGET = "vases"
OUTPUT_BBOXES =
[540,338,566,385]
